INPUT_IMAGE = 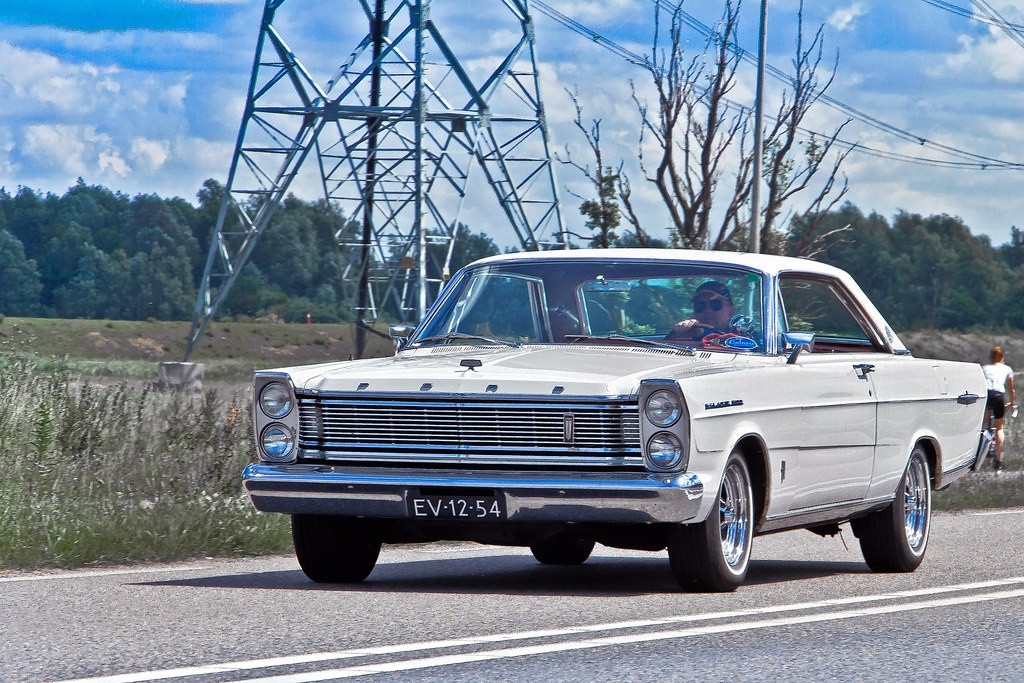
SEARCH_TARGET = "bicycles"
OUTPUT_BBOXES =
[972,403,1017,471]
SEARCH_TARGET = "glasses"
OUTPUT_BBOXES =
[694,299,729,313]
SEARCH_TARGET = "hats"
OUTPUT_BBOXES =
[694,281,733,303]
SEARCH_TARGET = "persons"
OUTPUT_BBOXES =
[661,281,762,354]
[978,345,1017,478]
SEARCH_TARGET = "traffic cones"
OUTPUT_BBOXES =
[305,314,312,323]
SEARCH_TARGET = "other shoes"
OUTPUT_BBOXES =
[994,460,1006,470]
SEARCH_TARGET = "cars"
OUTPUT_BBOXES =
[242,248,992,593]
